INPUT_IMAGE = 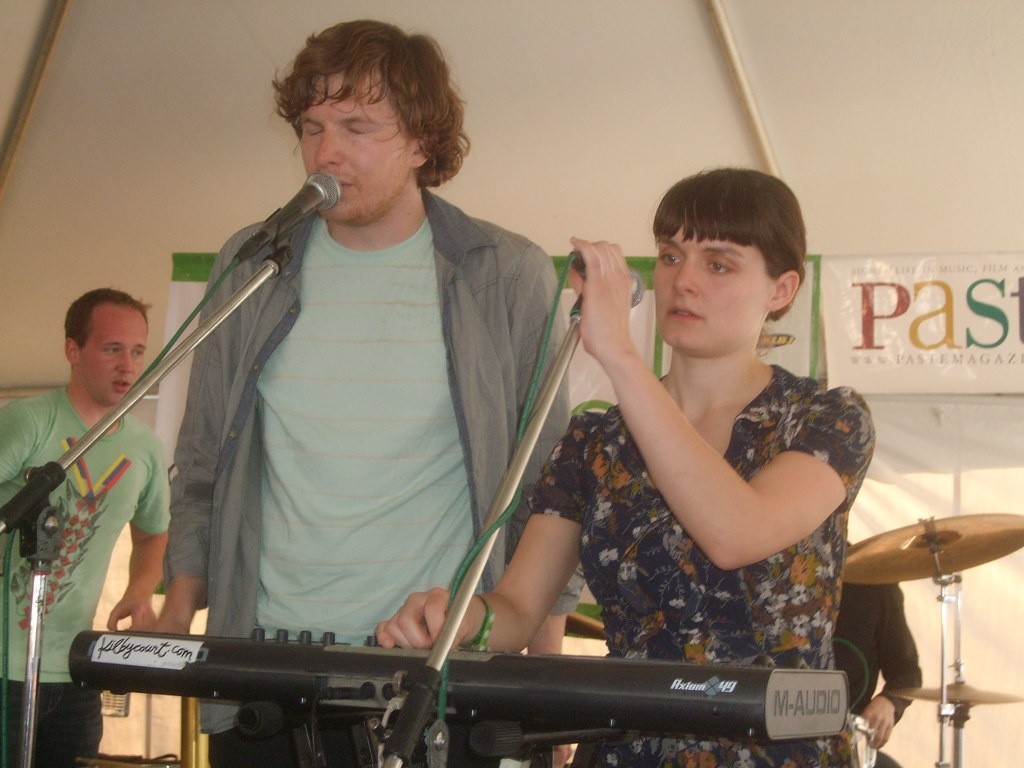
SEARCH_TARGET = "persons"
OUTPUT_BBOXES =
[0,286,170,768]
[154,20,570,768]
[374,169,923,768]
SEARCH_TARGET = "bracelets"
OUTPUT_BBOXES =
[457,593,496,653]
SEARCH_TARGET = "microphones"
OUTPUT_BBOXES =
[234,172,343,262]
[567,250,645,308]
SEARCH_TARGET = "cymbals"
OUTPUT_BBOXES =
[841,512,1024,587]
[886,681,1024,705]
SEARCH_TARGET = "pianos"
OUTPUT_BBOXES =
[66,628,853,747]
